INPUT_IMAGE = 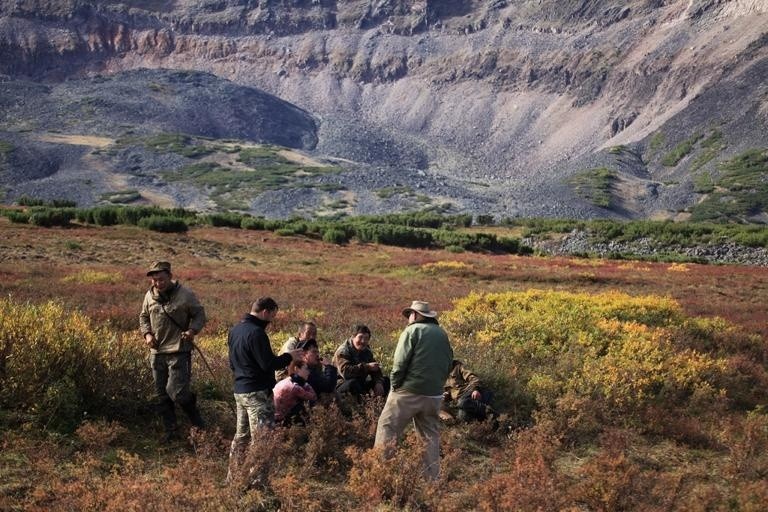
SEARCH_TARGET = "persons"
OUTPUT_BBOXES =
[331,325,389,421]
[373,300,453,482]
[138,260,206,444]
[225,295,306,496]
[270,322,338,448]
[441,359,519,448]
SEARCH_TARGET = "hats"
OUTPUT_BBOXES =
[146,259,171,277]
[401,300,438,318]
[295,338,317,353]
